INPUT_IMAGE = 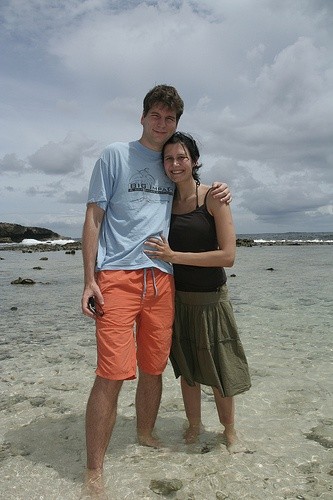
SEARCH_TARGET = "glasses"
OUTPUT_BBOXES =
[163,157,190,164]
[89,297,104,317]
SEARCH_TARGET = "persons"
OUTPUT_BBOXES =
[81,85,232,500]
[143,130,242,454]
